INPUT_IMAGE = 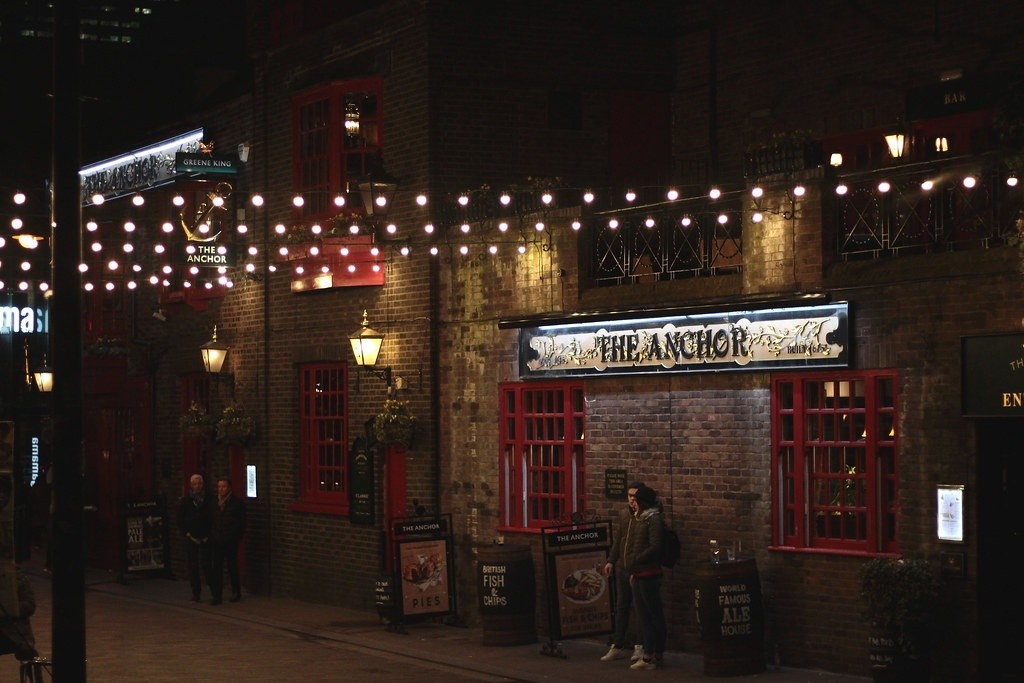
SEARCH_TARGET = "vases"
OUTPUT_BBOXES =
[184,425,207,439]
[516,188,581,212]
[741,148,822,181]
[86,355,126,367]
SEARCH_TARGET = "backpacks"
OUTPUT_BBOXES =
[647,514,680,569]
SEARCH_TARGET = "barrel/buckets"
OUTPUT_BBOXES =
[695,559,766,678]
[478,544,537,646]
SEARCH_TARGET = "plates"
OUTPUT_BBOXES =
[563,570,606,605]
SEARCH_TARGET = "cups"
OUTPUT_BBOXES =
[727,549,735,560]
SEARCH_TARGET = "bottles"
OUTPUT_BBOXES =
[592,560,602,576]
[710,539,719,564]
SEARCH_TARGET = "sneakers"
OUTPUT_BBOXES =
[631,645,644,662]
[600,644,627,661]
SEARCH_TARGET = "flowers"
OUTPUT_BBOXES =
[85,337,130,355]
[751,126,826,155]
[272,214,376,248]
[513,175,573,191]
[177,401,215,441]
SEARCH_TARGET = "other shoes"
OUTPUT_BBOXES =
[211,599,222,606]
[192,594,199,601]
[630,656,656,670]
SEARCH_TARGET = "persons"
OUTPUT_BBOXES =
[177,473,247,606]
[601,482,667,672]
[0,538,37,660]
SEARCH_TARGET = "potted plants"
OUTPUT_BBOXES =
[373,401,416,448]
[215,399,256,447]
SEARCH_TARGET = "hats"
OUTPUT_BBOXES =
[635,487,656,509]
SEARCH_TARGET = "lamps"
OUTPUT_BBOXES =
[347,309,392,400]
[195,203,212,226]
[936,484,964,543]
[345,98,360,148]
[199,325,234,384]
[858,556,931,683]
[34,353,55,398]
[883,119,907,162]
